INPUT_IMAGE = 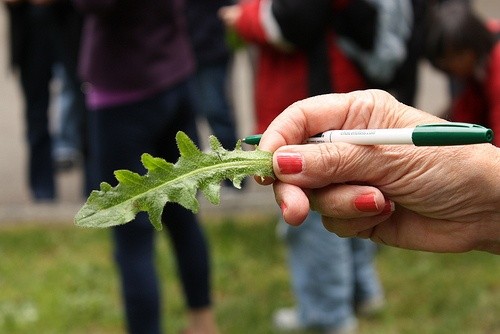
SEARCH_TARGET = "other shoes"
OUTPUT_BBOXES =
[273,308,358,333]
[355,294,385,315]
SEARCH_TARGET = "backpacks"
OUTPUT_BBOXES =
[332,0,415,82]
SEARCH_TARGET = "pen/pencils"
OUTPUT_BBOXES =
[240,122,494,147]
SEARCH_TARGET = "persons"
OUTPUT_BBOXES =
[219,0,386,334]
[0,0,89,201]
[249,88,500,256]
[175,0,249,191]
[60,0,223,334]
[417,0,500,148]
[330,1,443,110]
